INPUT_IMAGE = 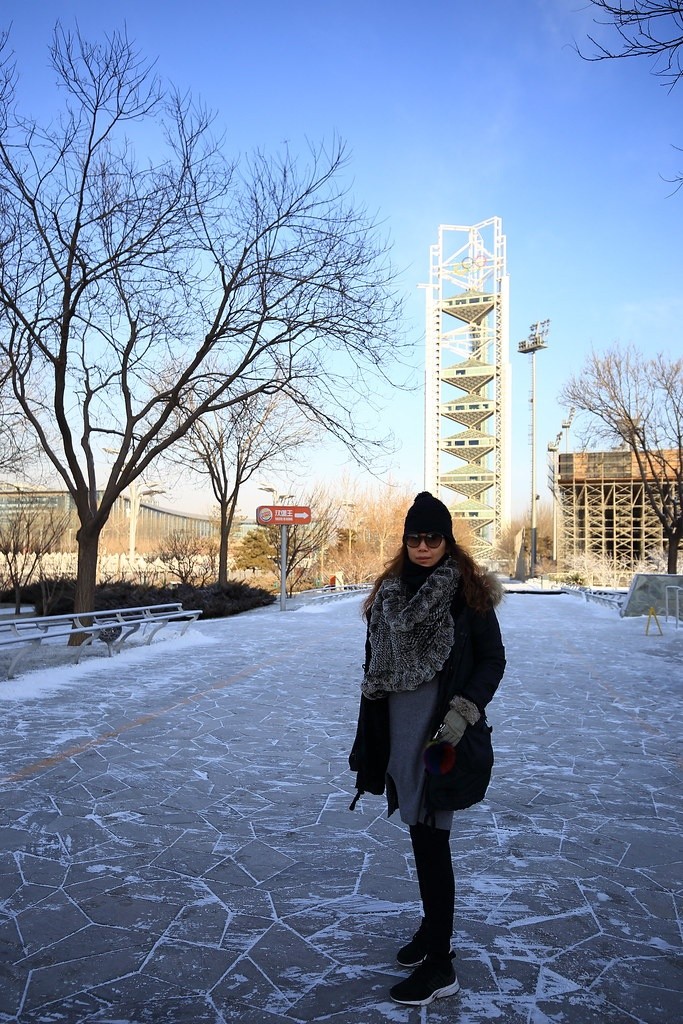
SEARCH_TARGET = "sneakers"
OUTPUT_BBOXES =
[389,950,460,1005]
[396,917,428,967]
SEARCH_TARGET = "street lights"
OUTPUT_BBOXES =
[517,319,550,578]
[128,482,167,567]
[343,501,355,552]
[548,432,563,561]
[257,482,297,507]
[563,406,575,453]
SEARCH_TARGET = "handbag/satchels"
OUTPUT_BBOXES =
[422,706,493,812]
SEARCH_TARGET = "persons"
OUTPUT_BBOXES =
[348,491,507,1006]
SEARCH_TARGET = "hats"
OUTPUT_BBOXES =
[404,491,456,545]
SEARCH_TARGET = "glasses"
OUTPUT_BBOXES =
[404,531,444,548]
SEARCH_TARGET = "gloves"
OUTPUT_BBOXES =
[426,709,468,752]
